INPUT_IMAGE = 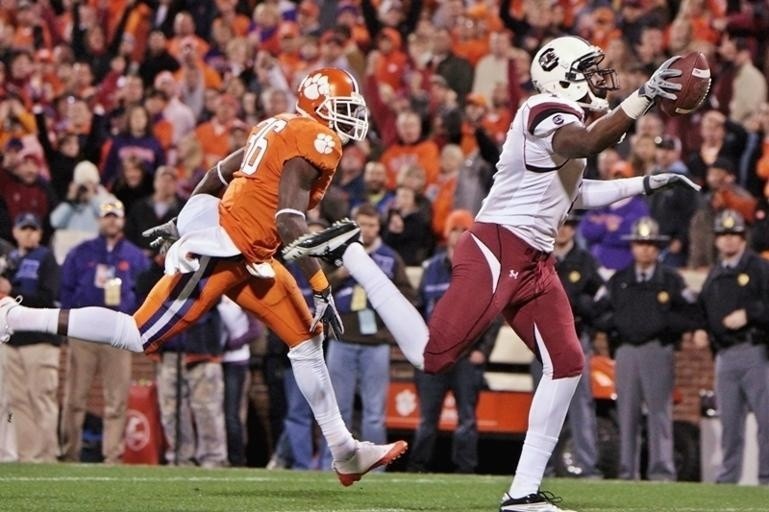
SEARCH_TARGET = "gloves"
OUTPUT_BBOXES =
[643,173,702,197]
[639,54,684,104]
[143,215,182,256]
[309,284,347,344]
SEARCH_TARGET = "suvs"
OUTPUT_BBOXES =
[385,325,700,481]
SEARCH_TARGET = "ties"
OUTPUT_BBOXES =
[641,270,648,285]
[725,264,732,274]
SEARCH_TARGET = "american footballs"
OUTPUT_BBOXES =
[657,52,713,118]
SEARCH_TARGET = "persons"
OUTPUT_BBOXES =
[0,65,412,490]
[275,35,704,511]
[0,2,769,483]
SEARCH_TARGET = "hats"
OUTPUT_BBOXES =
[95,197,126,218]
[14,211,43,232]
[440,208,475,235]
[614,214,673,245]
[70,159,102,189]
[709,209,748,235]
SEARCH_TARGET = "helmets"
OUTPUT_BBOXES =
[528,34,612,115]
[295,66,362,147]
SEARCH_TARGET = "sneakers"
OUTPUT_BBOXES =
[280,217,367,265]
[1,292,23,344]
[497,489,581,511]
[330,439,410,489]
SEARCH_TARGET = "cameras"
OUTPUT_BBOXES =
[78,186,88,194]
